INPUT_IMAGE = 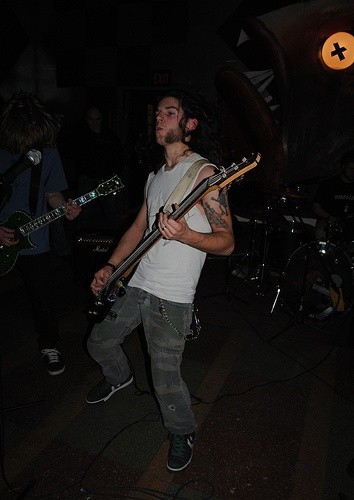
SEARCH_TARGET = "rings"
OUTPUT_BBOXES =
[162,226,167,232]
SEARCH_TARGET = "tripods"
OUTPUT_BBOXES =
[198,198,340,345]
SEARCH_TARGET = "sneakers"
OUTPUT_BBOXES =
[40,348,66,376]
[86,370,134,403]
[167,430,195,471]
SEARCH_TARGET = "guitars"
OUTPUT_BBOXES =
[0,171,126,277]
[88,153,262,324]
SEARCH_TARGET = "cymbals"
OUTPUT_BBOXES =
[261,190,304,199]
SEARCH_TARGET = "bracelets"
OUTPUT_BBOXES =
[105,263,116,273]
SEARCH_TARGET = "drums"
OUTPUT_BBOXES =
[281,241,354,323]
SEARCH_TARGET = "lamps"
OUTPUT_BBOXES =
[318,32,354,74]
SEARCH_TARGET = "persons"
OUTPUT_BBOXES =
[86,93,235,474]
[311,150,354,227]
[0,91,81,377]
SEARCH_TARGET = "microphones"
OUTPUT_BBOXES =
[0,149,42,195]
